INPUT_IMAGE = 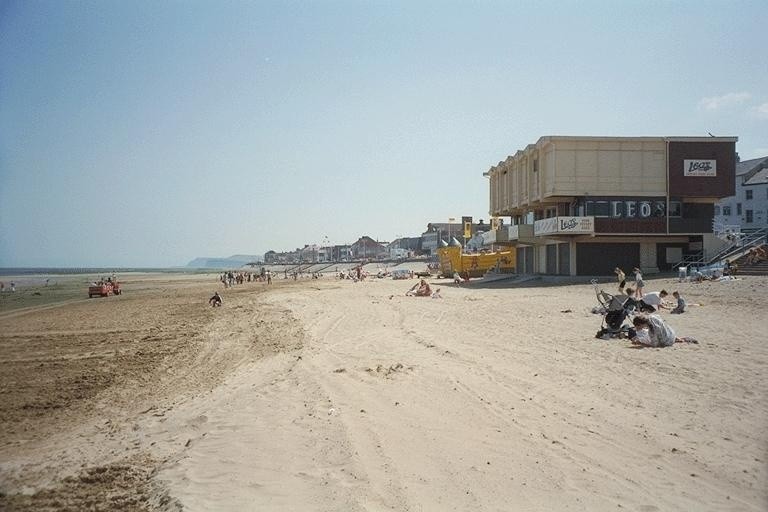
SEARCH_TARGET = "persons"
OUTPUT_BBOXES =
[47,278,49,285]
[415,279,442,299]
[744,246,766,265]
[209,292,223,307]
[614,266,686,347]
[453,269,470,284]
[724,259,738,274]
[0,280,15,292]
[92,277,111,287]
[215,265,413,288]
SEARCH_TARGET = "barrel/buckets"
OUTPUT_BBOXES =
[678,266,688,279]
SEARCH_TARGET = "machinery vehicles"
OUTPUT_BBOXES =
[89,281,122,298]
